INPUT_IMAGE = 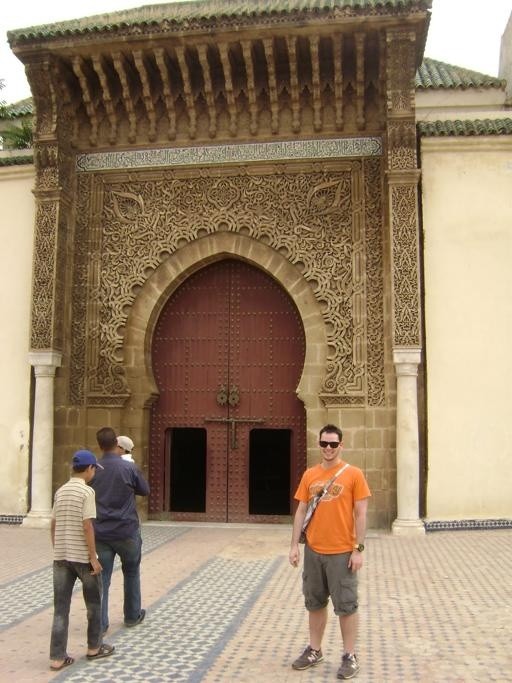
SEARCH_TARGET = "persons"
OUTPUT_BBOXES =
[87,427,150,636]
[50,451,115,669]
[288,424,371,679]
[115,434,135,463]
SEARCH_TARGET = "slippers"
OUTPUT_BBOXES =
[48,656,74,670]
[86,642,117,659]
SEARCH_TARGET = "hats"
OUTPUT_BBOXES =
[115,435,135,453]
[71,450,105,473]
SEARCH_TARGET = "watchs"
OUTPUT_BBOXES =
[353,544,364,552]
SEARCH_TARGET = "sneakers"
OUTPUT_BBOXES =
[123,609,146,628]
[291,645,325,671]
[335,652,362,681]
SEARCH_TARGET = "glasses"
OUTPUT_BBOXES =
[318,440,341,449]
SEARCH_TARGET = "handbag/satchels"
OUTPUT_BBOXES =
[299,530,309,544]
